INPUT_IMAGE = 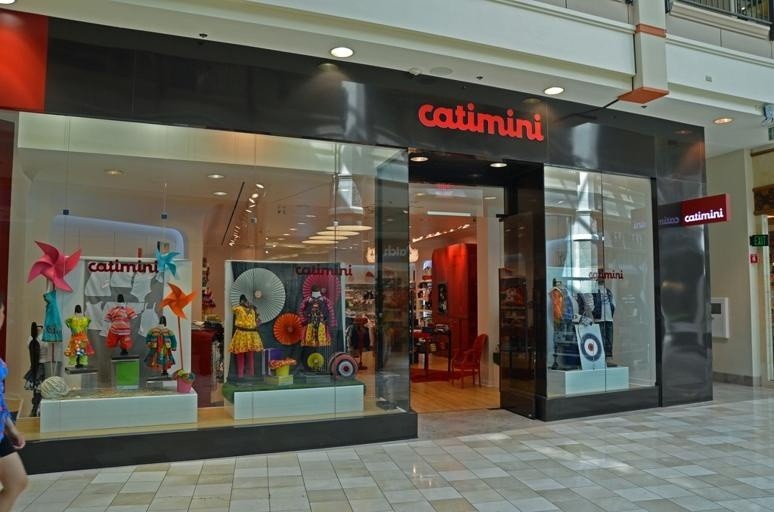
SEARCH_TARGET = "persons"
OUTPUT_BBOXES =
[590,279,618,365]
[299,285,337,372]
[103,294,137,355]
[228,295,264,379]
[63,305,94,367]
[143,316,177,374]
[0,299,28,511]
[550,281,578,346]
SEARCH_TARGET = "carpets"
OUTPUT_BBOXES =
[410,368,477,383]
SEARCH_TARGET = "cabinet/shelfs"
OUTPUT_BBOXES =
[417,281,433,327]
[432,242,475,358]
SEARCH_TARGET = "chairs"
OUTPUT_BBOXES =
[451,333,486,390]
